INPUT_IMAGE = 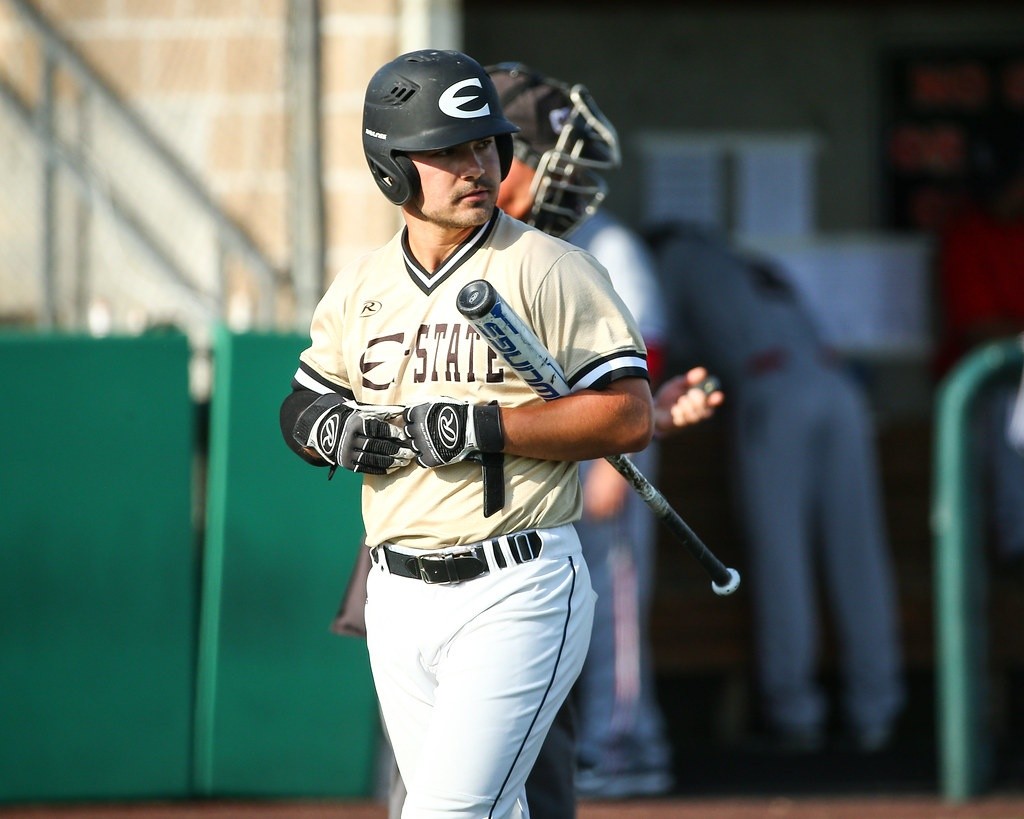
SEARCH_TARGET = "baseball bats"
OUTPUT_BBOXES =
[456,278,742,595]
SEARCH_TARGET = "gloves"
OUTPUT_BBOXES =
[388,396,506,468]
[291,392,417,481]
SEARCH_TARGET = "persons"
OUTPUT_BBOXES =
[280,50,654,819]
[389,64,723,819]
[563,215,668,797]
[648,220,904,748]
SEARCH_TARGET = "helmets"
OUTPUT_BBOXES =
[488,68,616,167]
[361,49,523,206]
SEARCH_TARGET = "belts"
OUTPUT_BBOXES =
[372,531,541,586]
